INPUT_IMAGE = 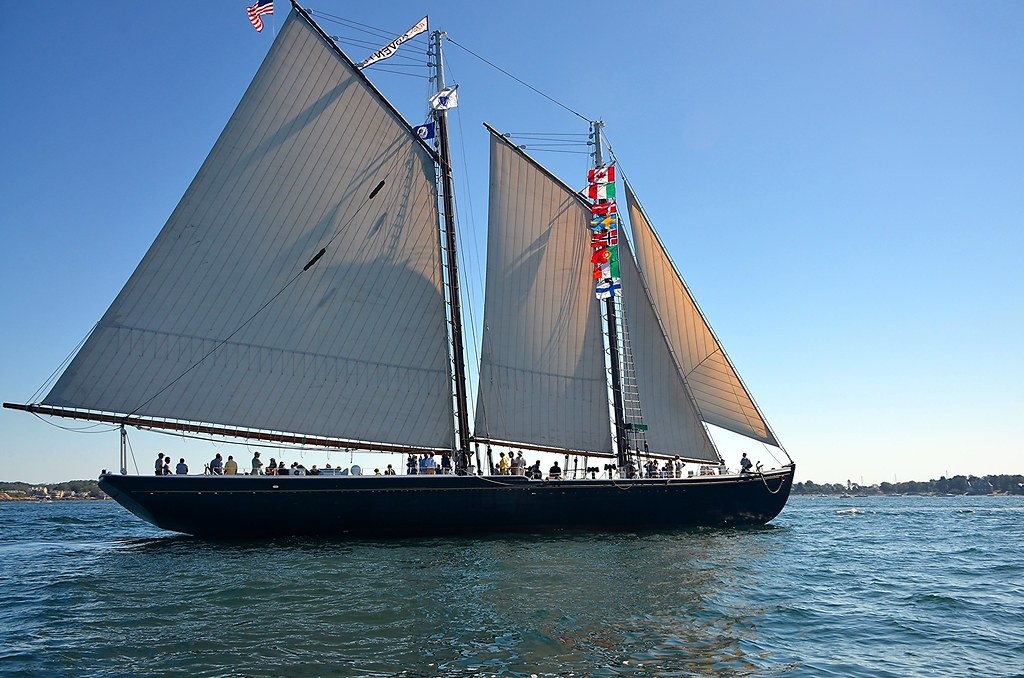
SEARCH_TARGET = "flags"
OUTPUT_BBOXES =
[413,122,435,140]
[588,167,622,299]
[429,88,458,109]
[246,0,274,33]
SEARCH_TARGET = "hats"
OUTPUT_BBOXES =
[517,451,523,455]
[430,451,435,454]
[158,453,165,456]
[254,451,261,455]
[269,458,275,462]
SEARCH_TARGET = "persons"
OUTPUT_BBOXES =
[550,461,561,479]
[337,466,342,473]
[740,453,753,471]
[155,453,172,475]
[250,452,285,475]
[210,453,237,475]
[701,466,715,475]
[496,451,542,479]
[406,447,468,475]
[385,464,396,475]
[374,469,381,475]
[176,458,188,475]
[623,454,685,478]
[291,462,319,475]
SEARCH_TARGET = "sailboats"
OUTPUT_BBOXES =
[2,0,797,546]
